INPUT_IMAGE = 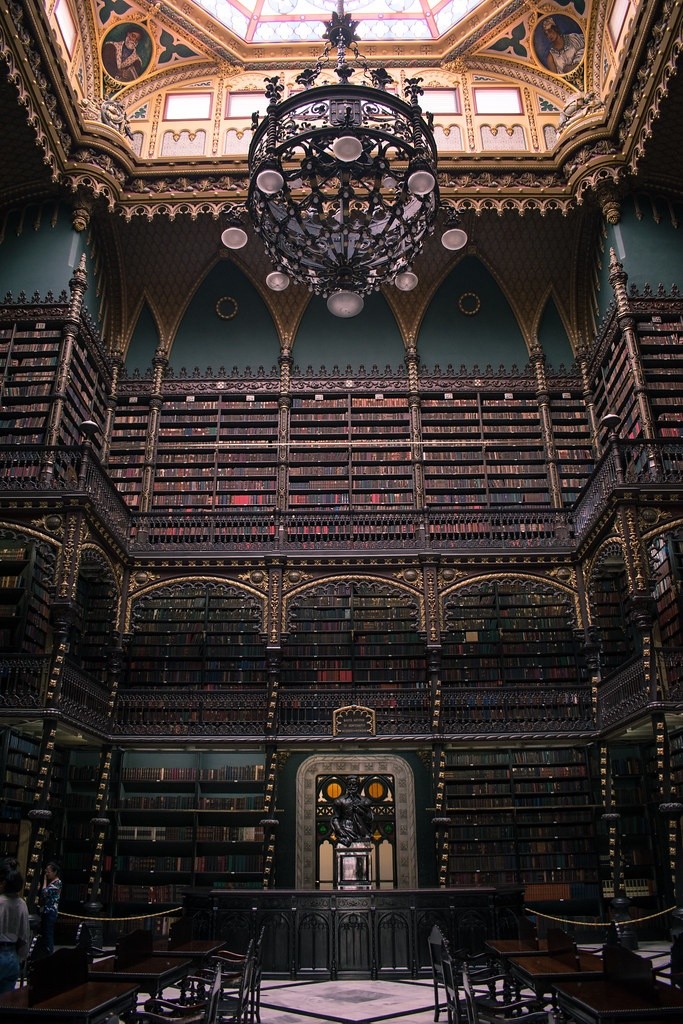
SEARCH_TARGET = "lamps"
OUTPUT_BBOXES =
[219,0,468,317]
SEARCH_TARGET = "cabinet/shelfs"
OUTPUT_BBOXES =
[0,245,683,979]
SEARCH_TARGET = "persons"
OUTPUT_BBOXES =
[38,861,62,955]
[0,857,21,882]
[0,870,31,995]
[333,774,373,847]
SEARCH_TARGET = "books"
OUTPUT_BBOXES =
[277,582,428,722]
[0,547,52,654]
[75,582,115,687]
[0,732,40,857]
[438,575,627,718]
[0,328,105,479]
[594,322,683,473]
[443,735,683,888]
[58,764,265,940]
[104,397,593,535]
[122,588,268,722]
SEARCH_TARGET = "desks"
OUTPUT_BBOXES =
[482,935,683,1024]
[0,937,227,1024]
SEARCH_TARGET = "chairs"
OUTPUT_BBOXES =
[119,923,269,1024]
[424,923,551,1024]
